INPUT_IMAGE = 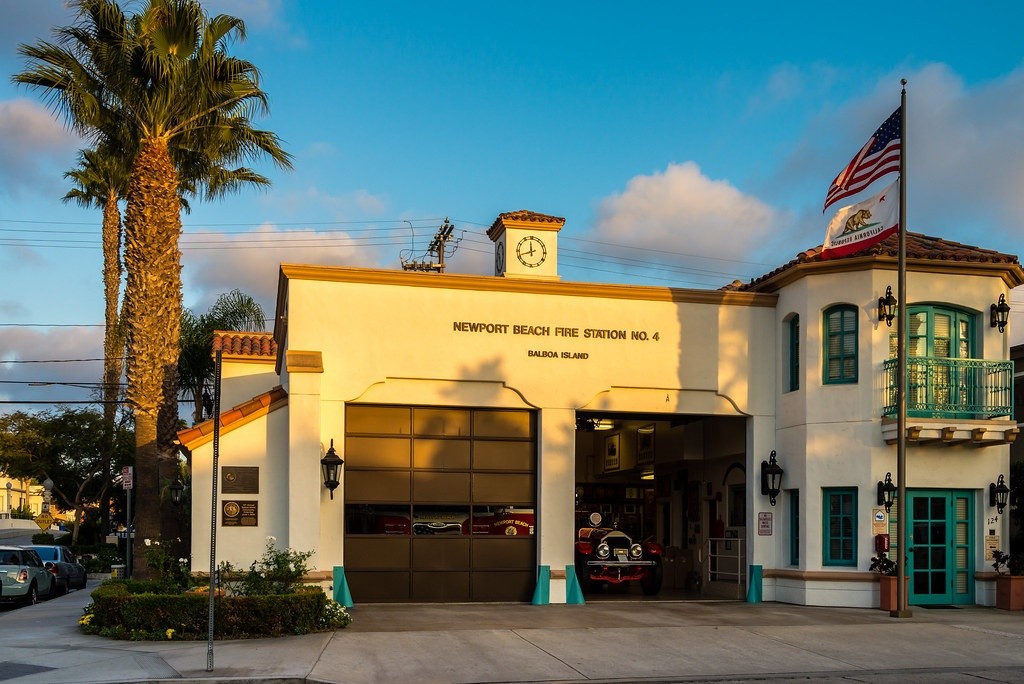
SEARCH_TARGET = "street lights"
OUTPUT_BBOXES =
[5,481,12,520]
[41,478,54,535]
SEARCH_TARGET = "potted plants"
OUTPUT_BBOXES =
[870,553,910,612]
[991,549,1023,611]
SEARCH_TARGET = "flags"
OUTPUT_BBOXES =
[823,106,905,215]
[816,178,903,261]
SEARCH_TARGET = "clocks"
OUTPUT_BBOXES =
[516,235,548,267]
[493,241,505,274]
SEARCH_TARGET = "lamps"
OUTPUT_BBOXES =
[876,472,897,515]
[878,285,897,327]
[320,440,345,500]
[989,474,1011,515]
[760,449,785,506]
[989,293,1010,333]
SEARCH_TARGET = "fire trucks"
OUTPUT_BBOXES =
[490,505,535,535]
[575,492,667,600]
[362,500,491,536]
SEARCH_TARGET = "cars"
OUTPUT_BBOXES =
[0,545,58,606]
[17,543,87,596]
